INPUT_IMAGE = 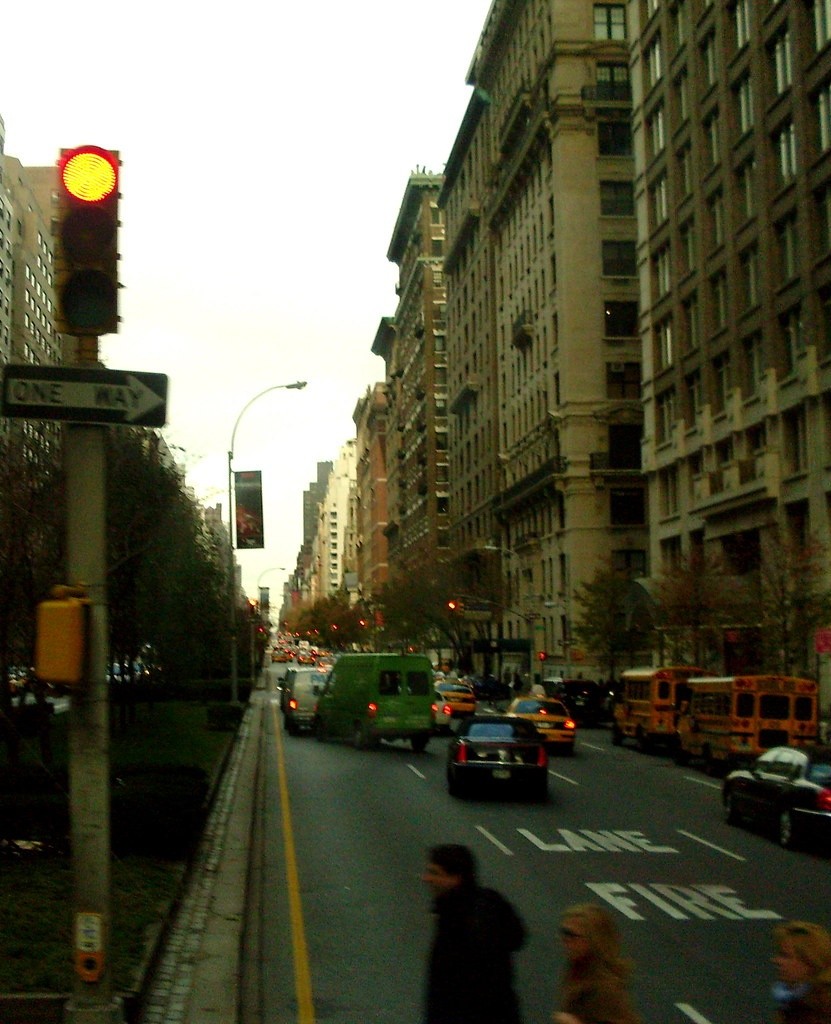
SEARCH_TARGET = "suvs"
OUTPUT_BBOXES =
[544,678,609,729]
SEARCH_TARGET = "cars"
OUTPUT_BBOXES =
[7,658,153,699]
[445,716,551,802]
[272,628,335,667]
[719,742,830,852]
[430,670,514,729]
[496,696,576,755]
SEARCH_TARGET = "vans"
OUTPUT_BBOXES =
[312,652,438,753]
[275,665,334,735]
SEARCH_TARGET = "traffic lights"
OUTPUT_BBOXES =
[446,595,467,620]
[54,140,121,337]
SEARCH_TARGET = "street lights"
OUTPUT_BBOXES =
[225,379,308,711]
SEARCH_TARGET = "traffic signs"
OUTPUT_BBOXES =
[1,363,169,428]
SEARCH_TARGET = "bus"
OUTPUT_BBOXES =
[673,673,818,778]
[605,668,716,756]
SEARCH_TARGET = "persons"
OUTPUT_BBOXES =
[421,843,529,1024]
[772,921,831,1024]
[435,658,626,708]
[548,904,645,1024]
